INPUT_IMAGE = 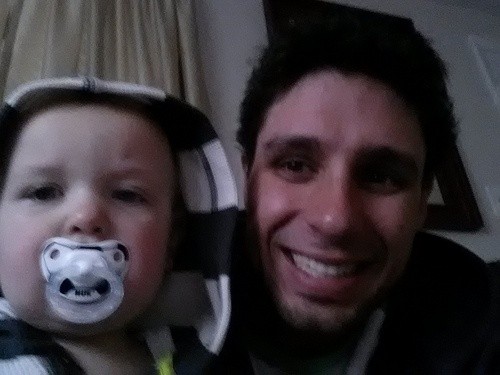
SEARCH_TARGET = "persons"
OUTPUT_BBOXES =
[228,0,500,375]
[0,76,236,375]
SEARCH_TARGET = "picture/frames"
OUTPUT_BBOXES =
[262,0,483,232]
